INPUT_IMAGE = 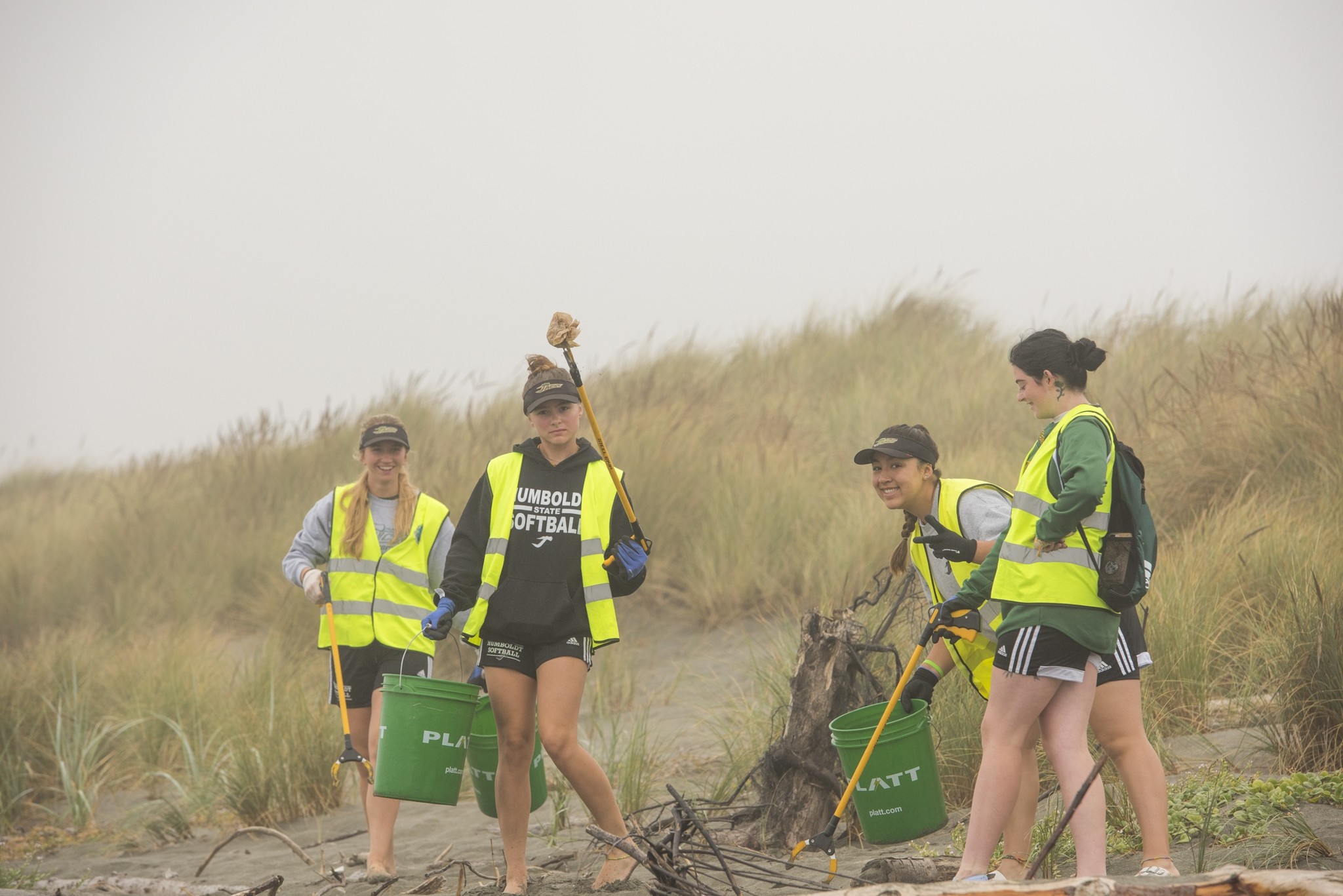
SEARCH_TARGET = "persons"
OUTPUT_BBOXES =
[417,352,649,895]
[279,414,457,885]
[868,422,1181,885]
[930,326,1118,883]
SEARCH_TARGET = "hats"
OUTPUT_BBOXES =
[853,432,936,467]
[522,378,582,418]
[359,422,409,451]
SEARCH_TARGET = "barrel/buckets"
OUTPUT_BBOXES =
[372,623,482,806]
[467,695,548,817]
[829,699,949,845]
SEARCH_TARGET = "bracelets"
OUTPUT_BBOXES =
[923,660,944,679]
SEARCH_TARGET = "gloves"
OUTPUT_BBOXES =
[602,535,648,585]
[932,595,968,645]
[421,598,456,641]
[899,667,940,713]
[301,569,330,604]
[912,514,978,566]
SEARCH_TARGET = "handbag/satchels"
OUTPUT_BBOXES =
[1050,406,1158,611]
